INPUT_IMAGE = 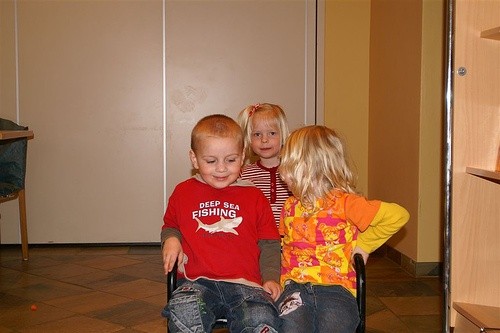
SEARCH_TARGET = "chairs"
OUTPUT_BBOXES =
[0,118,34,260]
[161,252,366,333]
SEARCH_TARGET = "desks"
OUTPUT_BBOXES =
[0,130,33,140]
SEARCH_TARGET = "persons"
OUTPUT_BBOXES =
[161,114,283,333]
[275,125,410,333]
[234,103,294,228]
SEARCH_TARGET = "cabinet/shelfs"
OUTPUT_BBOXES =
[451,0,500,333]
